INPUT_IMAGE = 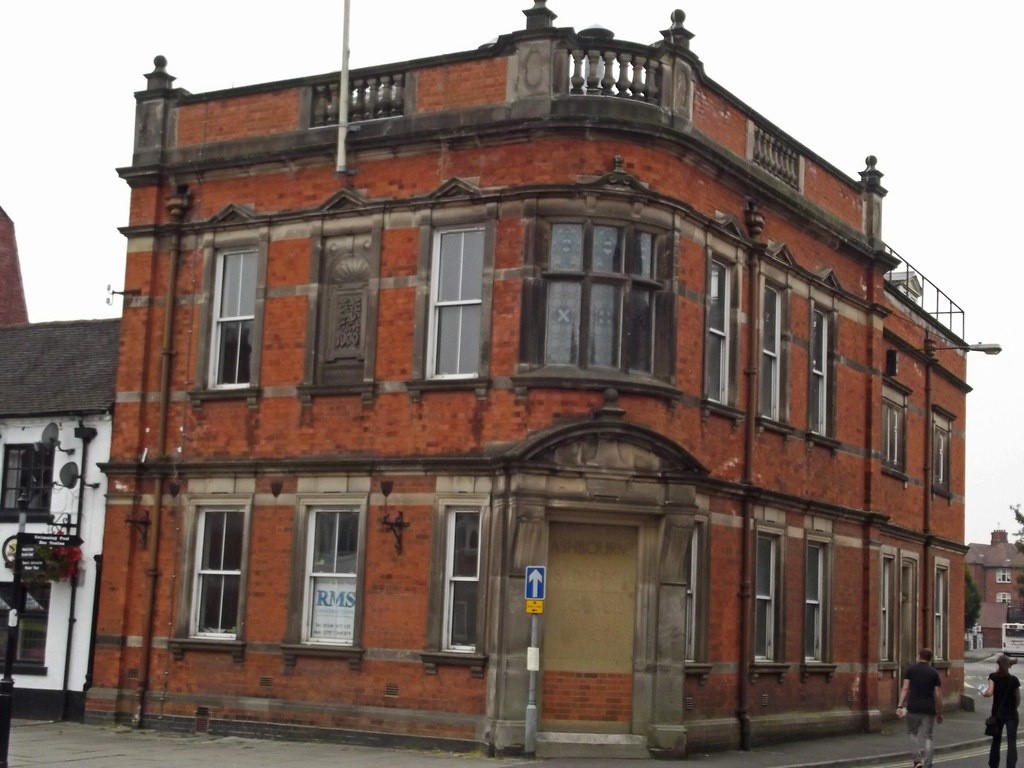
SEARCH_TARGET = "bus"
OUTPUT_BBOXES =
[1002,623,1024,656]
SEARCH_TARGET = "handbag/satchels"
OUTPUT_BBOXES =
[984,716,993,736]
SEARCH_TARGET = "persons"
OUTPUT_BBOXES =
[895,648,944,768]
[981,655,1021,768]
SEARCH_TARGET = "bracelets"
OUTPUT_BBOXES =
[897,706,903,709]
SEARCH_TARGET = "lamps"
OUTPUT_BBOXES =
[39,422,76,456]
[59,462,101,489]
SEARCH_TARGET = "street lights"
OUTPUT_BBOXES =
[920,329,1003,644]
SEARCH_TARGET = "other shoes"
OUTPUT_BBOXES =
[914,761,922,768]
